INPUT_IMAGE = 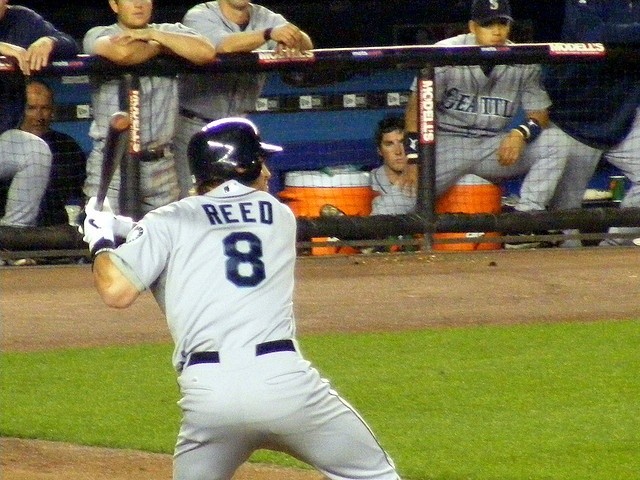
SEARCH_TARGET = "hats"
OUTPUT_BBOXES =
[471,0,514,25]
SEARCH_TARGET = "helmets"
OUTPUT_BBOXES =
[188,117,282,190]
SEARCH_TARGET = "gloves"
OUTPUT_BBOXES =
[75,196,133,257]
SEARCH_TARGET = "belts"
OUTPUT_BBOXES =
[187,339,294,364]
[140,147,163,161]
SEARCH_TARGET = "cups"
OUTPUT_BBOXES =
[609,176,627,203]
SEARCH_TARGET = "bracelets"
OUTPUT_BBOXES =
[403,131,420,165]
[509,117,542,142]
[263,27,273,42]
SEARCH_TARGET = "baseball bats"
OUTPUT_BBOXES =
[78,112,132,235]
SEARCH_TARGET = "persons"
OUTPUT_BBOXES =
[83,0,216,222]
[319,0,574,249]
[0,76,88,251]
[0,1,82,266]
[168,0,314,200]
[371,116,419,199]
[78,117,400,479]
[539,1,640,247]
